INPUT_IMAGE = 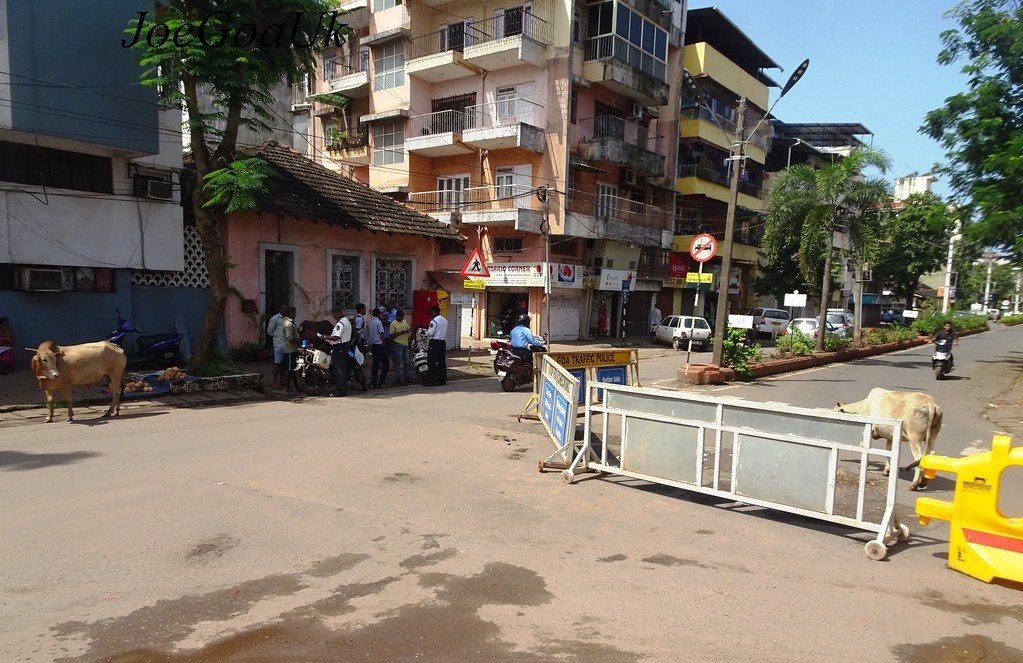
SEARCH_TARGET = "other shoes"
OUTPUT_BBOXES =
[372,385,380,389]
[330,391,346,397]
[397,379,405,383]
[381,383,392,388]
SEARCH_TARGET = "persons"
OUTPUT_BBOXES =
[510,315,546,382]
[370,301,398,366]
[353,303,371,379]
[321,308,352,397]
[389,310,416,382]
[267,305,288,389]
[368,308,391,388]
[928,321,958,374]
[283,307,303,393]
[0,317,16,374]
[420,306,447,386]
[651,304,662,339]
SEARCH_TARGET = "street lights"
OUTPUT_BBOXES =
[683,58,810,370]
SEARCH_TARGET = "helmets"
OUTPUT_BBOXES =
[358,338,371,353]
[518,315,531,327]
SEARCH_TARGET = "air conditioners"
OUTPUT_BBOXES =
[628,101,643,118]
[620,167,637,186]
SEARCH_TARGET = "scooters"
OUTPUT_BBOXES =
[409,321,431,383]
[99,306,185,370]
[931,338,955,380]
[491,331,550,392]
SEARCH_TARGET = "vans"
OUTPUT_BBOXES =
[880,307,904,325]
[743,307,789,339]
[816,308,854,338]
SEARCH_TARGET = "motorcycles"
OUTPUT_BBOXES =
[291,327,368,396]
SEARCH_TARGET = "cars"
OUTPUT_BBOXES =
[927,309,1023,320]
[782,315,841,352]
[649,315,712,351]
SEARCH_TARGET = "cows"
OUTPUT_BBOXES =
[24,340,127,422]
[831,386,943,491]
